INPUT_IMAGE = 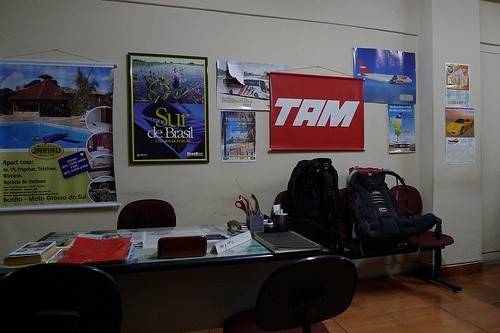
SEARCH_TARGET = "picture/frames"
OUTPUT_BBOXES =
[128,52,210,164]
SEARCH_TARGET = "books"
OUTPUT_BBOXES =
[3,241,62,266]
[253,231,321,255]
[44,232,146,264]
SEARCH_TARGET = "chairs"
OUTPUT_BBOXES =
[117,199,176,230]
[223,255,358,333]
[0,263,123,333]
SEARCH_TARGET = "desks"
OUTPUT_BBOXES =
[0,225,329,333]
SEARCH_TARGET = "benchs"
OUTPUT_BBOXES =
[275,185,462,293]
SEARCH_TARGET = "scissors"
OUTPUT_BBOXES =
[235,195,251,214]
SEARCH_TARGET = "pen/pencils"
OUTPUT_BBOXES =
[245,203,256,214]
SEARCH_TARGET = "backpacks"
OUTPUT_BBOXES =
[286,158,342,245]
[348,166,436,243]
